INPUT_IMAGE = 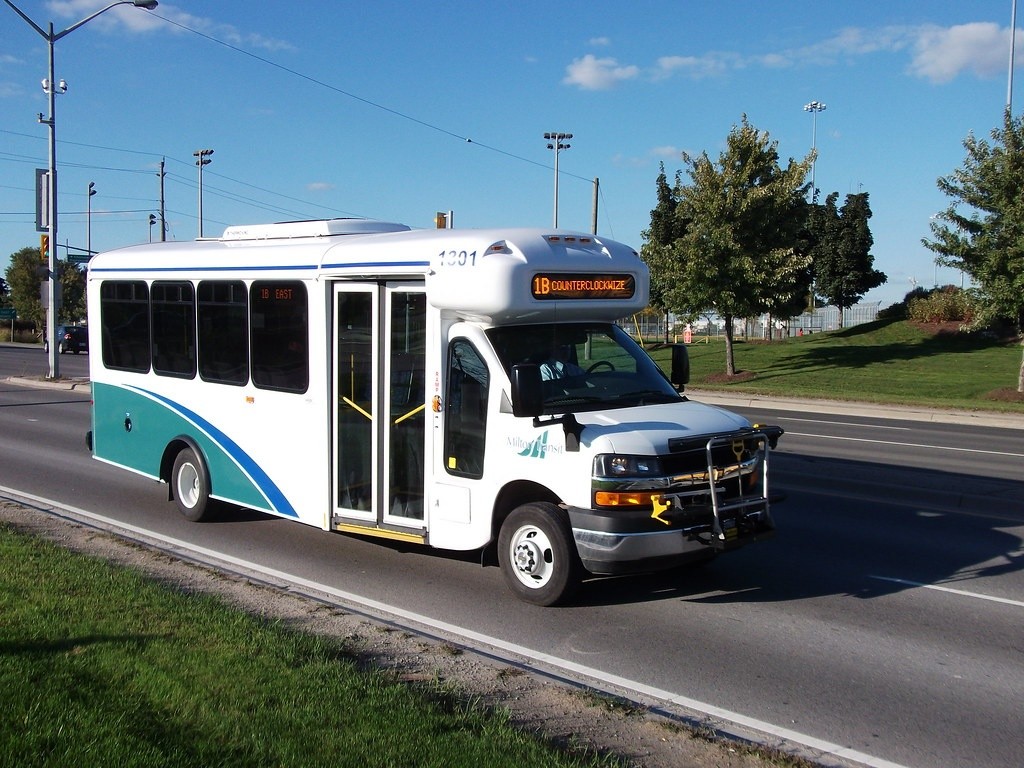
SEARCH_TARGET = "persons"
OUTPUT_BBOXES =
[808,328,813,334]
[624,322,631,336]
[539,340,590,398]
[741,322,746,337]
[798,328,803,337]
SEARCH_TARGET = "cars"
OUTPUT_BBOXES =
[44,325,89,355]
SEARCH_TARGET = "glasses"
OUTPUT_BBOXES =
[560,344,570,350]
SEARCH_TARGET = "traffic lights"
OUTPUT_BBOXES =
[434,212,447,229]
[41,234,50,262]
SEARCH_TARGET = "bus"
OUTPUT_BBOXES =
[84,219,787,607]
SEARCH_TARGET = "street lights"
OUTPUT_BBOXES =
[193,148,214,238]
[0,0,161,379]
[87,181,96,258]
[544,131,577,229]
[802,101,828,335]
[149,213,156,243]
